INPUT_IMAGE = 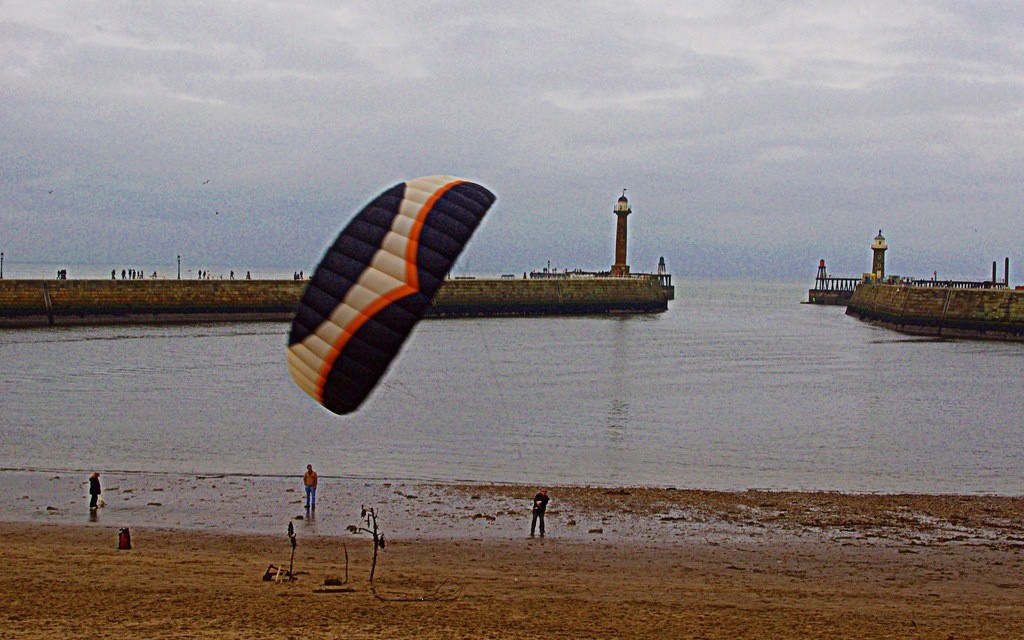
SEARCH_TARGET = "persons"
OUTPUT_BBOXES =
[527,487,549,539]
[189,270,223,280]
[523,271,526,279]
[245,270,251,280]
[111,268,159,280]
[304,464,319,509]
[537,272,540,279]
[229,269,234,279]
[294,270,303,281]
[530,271,534,279]
[87,470,101,509]
[57,268,67,279]
[542,267,582,274]
